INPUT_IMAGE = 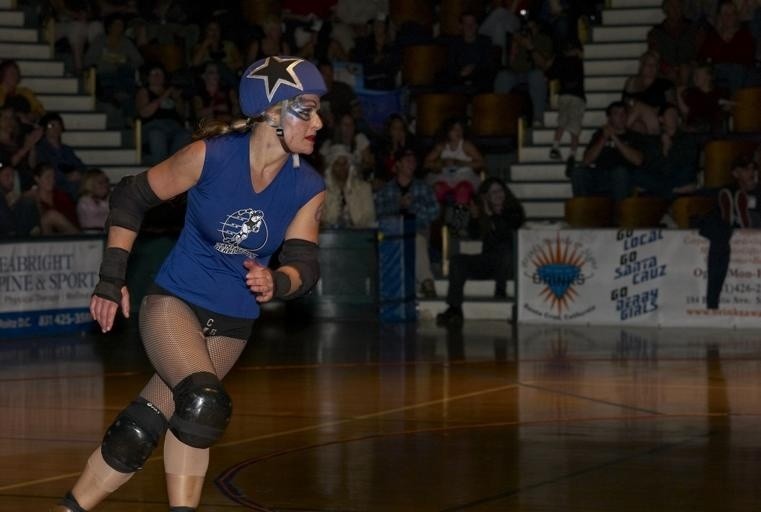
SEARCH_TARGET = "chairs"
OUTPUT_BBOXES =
[565,89,760,226]
[136,0,558,164]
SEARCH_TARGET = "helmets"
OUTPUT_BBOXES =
[240,55,327,117]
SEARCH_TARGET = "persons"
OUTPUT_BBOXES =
[25,1,604,229]
[58,55,329,512]
[573,0,759,226]
[380,119,486,296]
[1,58,89,242]
[436,178,526,324]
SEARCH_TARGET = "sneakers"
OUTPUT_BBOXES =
[549,149,575,178]
[495,282,507,296]
[716,189,752,231]
[438,310,464,321]
[421,279,436,299]
